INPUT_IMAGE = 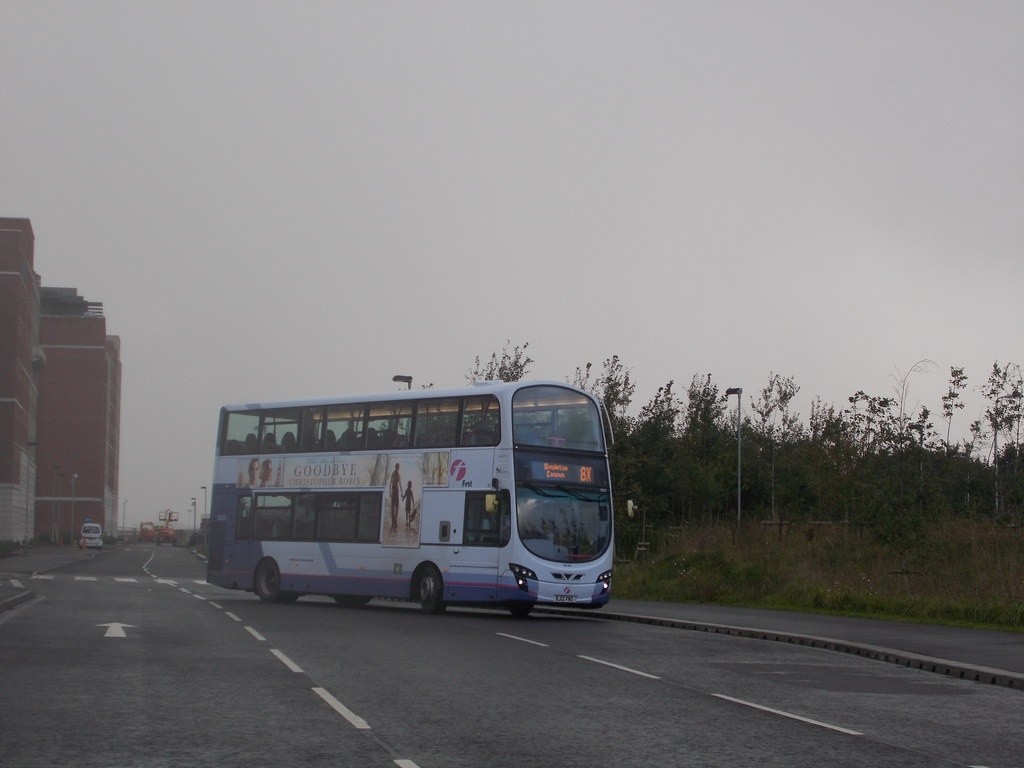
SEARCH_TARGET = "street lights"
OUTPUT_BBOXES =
[120,496,127,545]
[23,441,78,547]
[188,485,208,548]
[726,388,747,541]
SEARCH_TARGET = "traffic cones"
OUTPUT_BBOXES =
[80,538,89,554]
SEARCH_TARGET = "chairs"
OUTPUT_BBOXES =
[229,427,408,454]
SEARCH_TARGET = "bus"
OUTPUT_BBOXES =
[205,380,638,619]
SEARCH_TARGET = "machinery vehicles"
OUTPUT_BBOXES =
[139,508,182,546]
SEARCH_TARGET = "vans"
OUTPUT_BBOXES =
[80,523,104,550]
